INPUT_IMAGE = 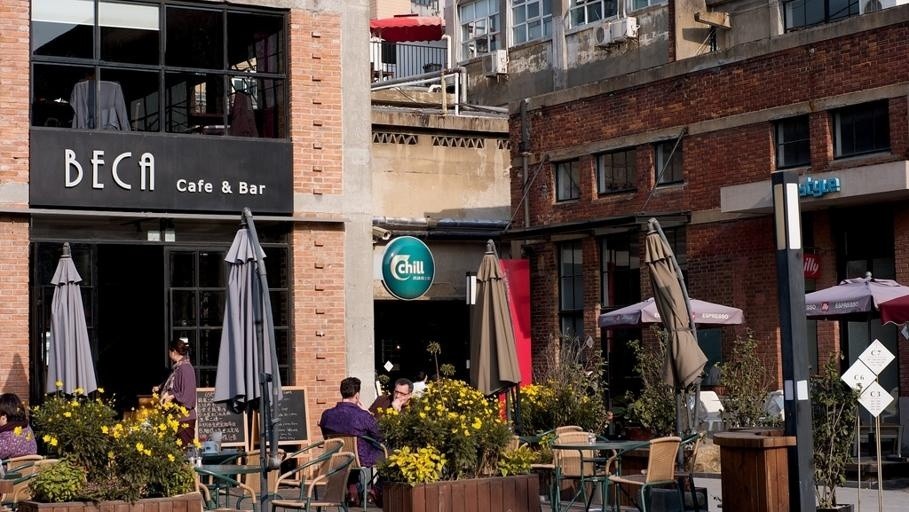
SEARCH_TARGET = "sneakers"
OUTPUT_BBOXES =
[349,486,361,507]
[370,485,383,508]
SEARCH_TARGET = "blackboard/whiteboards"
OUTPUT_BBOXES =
[257,386,311,446]
[194,388,249,447]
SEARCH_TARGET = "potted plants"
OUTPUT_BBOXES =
[808,349,855,511]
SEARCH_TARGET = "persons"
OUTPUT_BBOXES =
[411,370,430,400]
[368,374,417,420]
[319,376,390,507]
[0,392,39,476]
[158,336,198,458]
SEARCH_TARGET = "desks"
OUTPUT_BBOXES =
[194,465,281,510]
[69,79,133,132]
[852,422,905,458]
[192,448,243,484]
[550,441,652,511]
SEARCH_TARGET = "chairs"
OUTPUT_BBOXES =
[2,455,60,510]
[489,426,707,511]
[192,433,389,511]
[188,89,260,137]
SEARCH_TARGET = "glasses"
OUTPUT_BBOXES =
[394,390,408,397]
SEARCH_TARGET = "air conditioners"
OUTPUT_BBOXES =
[590,21,613,51]
[478,44,513,79]
[609,16,638,42]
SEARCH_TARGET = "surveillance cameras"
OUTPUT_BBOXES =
[373,225,391,241]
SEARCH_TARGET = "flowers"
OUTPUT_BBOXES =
[11,381,205,504]
[509,381,615,435]
[374,377,540,489]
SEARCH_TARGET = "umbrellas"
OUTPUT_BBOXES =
[799,269,909,434]
[207,204,285,468]
[469,237,528,437]
[39,236,98,401]
[641,215,710,476]
[596,285,744,343]
